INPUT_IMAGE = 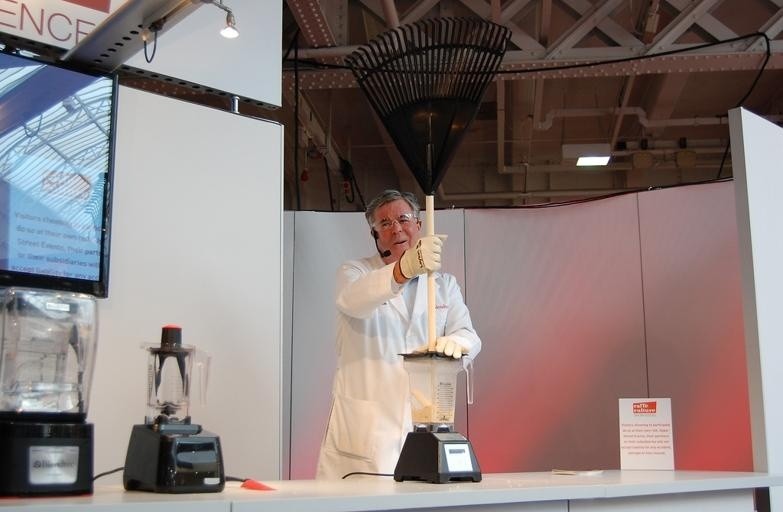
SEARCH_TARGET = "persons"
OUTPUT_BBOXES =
[315,189,482,481]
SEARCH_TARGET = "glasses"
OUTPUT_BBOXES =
[375,213,415,231]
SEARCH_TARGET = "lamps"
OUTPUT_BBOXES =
[562,144,612,166]
[220,11,241,40]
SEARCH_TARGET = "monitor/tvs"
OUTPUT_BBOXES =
[0,48,119,300]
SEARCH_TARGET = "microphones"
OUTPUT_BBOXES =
[372,231,392,259]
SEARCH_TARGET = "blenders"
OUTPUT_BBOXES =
[0,285,99,498]
[122,324,225,492]
[390,351,481,482]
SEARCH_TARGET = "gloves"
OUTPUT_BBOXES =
[412,335,470,361]
[399,233,447,279]
